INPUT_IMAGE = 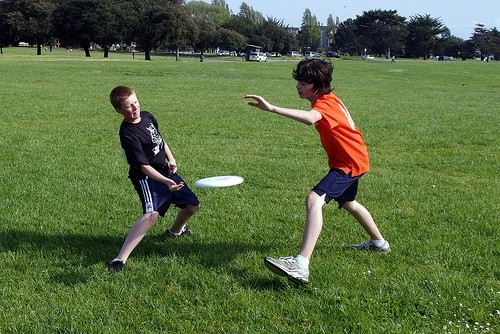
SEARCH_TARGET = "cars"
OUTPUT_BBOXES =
[249,52,267,62]
[217,49,281,57]
[305,51,319,57]
[287,51,302,57]
[326,52,340,58]
[363,55,375,60]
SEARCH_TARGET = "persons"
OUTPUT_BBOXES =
[49,43,53,52]
[480,54,490,62]
[64,45,74,53]
[244,58,391,284]
[433,54,452,61]
[108,86,201,268]
[391,55,396,63]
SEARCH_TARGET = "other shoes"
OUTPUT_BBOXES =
[109,260,126,272]
[162,229,190,238]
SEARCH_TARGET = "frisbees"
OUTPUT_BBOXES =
[194,176,244,188]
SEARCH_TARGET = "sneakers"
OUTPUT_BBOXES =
[351,238,390,252]
[264,256,310,286]
